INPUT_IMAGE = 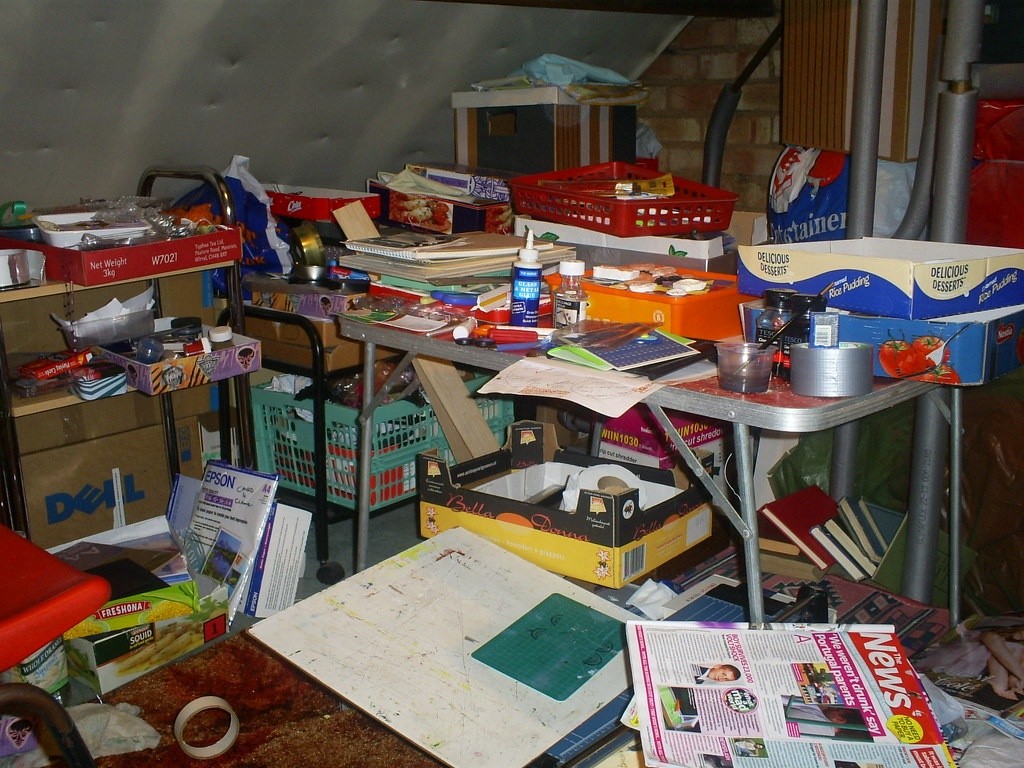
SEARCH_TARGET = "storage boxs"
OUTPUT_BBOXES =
[45,514,200,639]
[406,160,512,203]
[451,86,638,172]
[548,264,760,341]
[21,416,204,552]
[416,419,717,589]
[510,161,741,237]
[166,473,312,617]
[245,366,516,509]
[245,313,399,368]
[737,297,1024,386]
[65,573,232,695]
[593,405,738,522]
[0,523,113,759]
[57,309,158,349]
[261,183,383,223]
[735,236,1024,323]
[0,223,244,287]
[92,314,264,395]
[365,178,514,237]
[534,403,592,446]
[13,382,218,453]
[31,211,153,251]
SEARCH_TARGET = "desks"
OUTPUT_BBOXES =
[339,317,967,629]
[101,524,646,768]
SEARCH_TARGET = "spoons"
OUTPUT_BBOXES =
[925,324,972,370]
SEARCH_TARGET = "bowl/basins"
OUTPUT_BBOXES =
[60,308,157,349]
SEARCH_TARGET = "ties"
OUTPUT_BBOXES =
[697,679,705,682]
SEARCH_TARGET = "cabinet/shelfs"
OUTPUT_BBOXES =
[217,303,533,590]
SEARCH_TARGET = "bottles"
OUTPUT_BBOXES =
[755,288,799,377]
[512,229,542,327]
[12,531,72,707]
[552,261,588,330]
[209,326,233,352]
[781,295,829,380]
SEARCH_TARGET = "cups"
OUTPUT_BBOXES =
[714,342,779,392]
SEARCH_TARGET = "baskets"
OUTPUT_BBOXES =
[510,161,740,239]
[248,372,516,514]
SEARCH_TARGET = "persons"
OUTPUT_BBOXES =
[913,623,1024,701]
[690,662,741,687]
[784,699,859,736]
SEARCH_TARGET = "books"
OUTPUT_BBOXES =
[339,228,577,298]
[627,484,1024,768]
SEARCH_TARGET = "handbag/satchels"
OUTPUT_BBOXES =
[184,155,295,275]
